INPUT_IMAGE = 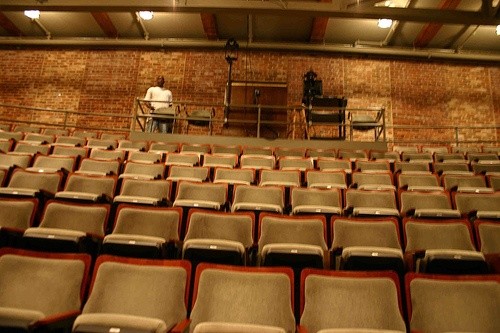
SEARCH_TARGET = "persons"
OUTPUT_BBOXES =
[145,75,173,134]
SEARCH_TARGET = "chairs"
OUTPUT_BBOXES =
[186,262,297,333]
[149,105,180,134]
[404,273,500,333]
[0,122,500,274]
[301,97,348,140]
[0,247,91,333]
[348,111,383,141]
[297,268,407,333]
[184,105,215,136]
[72,254,192,333]
